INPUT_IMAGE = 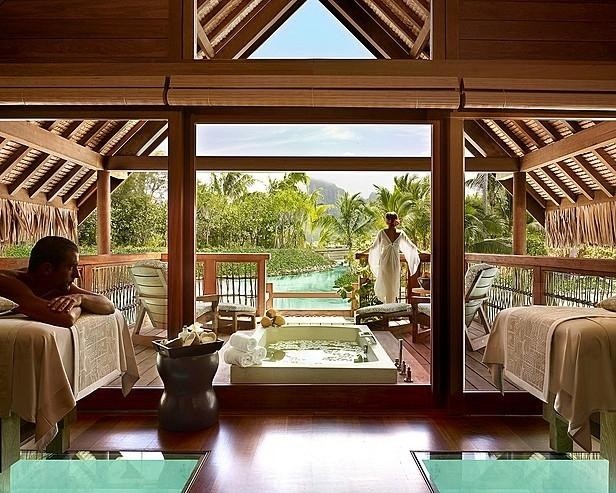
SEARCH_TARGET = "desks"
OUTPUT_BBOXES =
[153,351,221,432]
[410,287,431,297]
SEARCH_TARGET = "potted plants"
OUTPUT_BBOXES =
[417,271,431,290]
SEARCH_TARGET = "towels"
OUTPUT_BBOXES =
[223,331,269,369]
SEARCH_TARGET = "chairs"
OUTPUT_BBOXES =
[353,260,499,353]
[126,256,256,349]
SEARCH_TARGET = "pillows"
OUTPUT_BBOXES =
[0,293,18,315]
[596,294,615,311]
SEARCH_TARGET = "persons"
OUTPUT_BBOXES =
[1,234,116,327]
[357,210,431,305]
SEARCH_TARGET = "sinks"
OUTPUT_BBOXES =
[229,323,396,384]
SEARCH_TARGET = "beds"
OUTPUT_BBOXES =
[481,303,616,461]
[2,305,141,472]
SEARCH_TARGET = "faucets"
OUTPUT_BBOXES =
[358,329,379,345]
